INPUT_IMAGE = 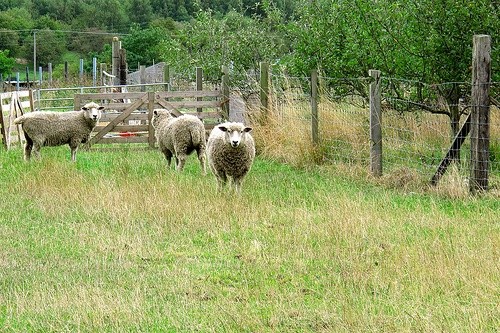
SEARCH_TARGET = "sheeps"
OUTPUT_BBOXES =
[14,102,105,161]
[151,108,206,177]
[206,122,255,195]
